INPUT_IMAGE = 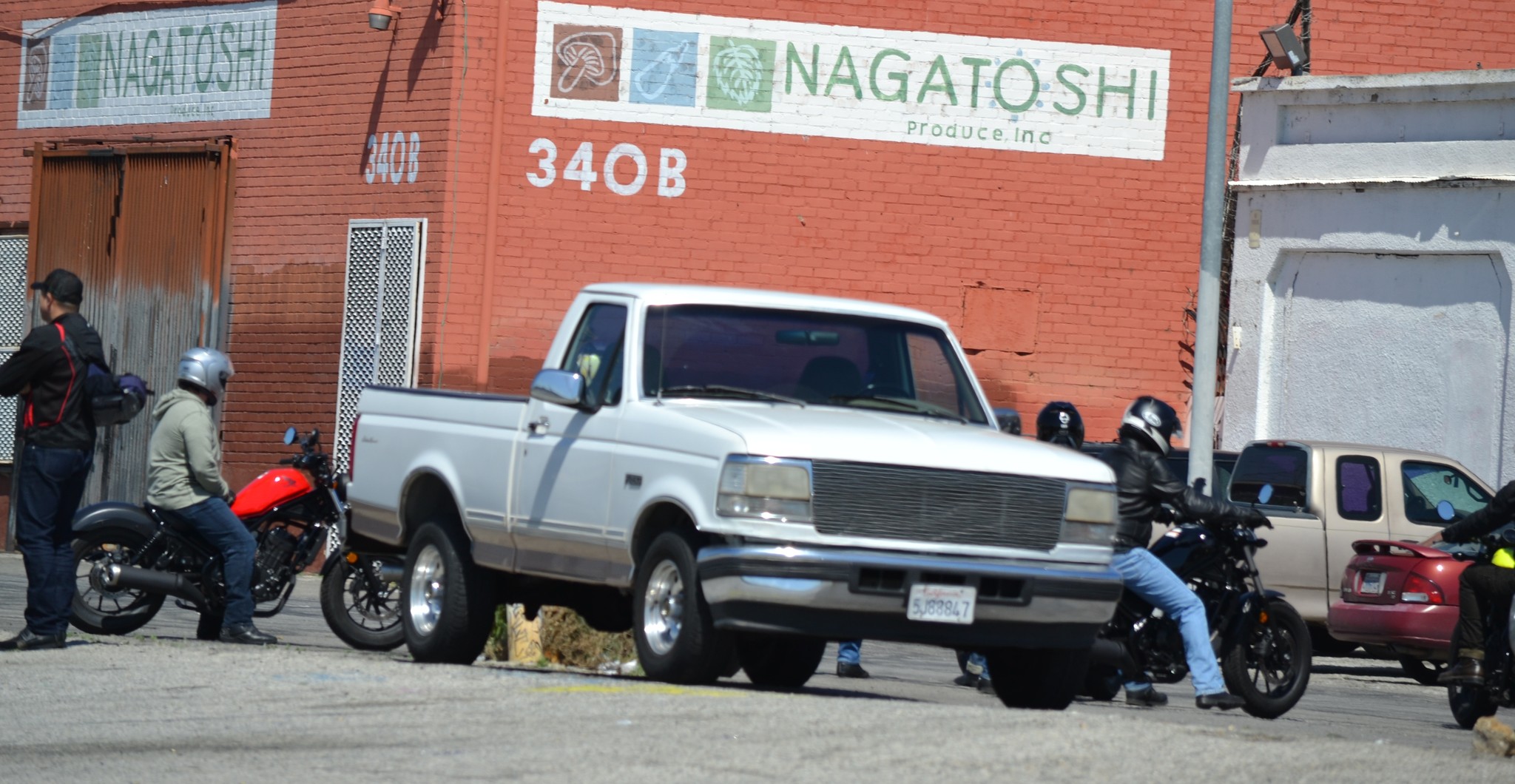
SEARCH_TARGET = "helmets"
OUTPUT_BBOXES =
[1035,401,1084,449]
[178,347,234,406]
[1122,395,1183,458]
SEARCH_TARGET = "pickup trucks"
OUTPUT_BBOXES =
[342,285,1123,712]
[1151,437,1497,661]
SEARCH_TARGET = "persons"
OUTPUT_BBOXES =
[954,401,1085,693]
[0,268,108,650]
[1429,481,1515,703]
[145,349,277,645]
[836,642,869,677]
[1100,396,1270,709]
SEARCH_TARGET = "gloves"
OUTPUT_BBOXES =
[220,490,236,508]
[1172,511,1193,526]
[1233,506,1272,531]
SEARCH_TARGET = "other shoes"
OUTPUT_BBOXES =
[953,674,978,687]
[1126,686,1167,706]
[837,662,869,678]
[976,676,994,694]
[1436,663,1483,685]
[1195,691,1245,711]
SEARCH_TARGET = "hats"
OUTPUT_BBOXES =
[29,268,83,304]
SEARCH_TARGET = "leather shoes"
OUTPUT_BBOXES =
[0,627,67,651]
[197,618,223,640]
[219,623,277,644]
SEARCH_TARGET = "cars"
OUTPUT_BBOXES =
[1324,520,1485,683]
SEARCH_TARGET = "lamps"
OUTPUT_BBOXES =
[368,0,402,30]
[1258,24,1309,76]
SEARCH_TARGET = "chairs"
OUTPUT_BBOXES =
[801,356,861,404]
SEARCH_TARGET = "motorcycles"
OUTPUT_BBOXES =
[1093,476,1312,718]
[1435,499,1515,729]
[65,427,409,652]
